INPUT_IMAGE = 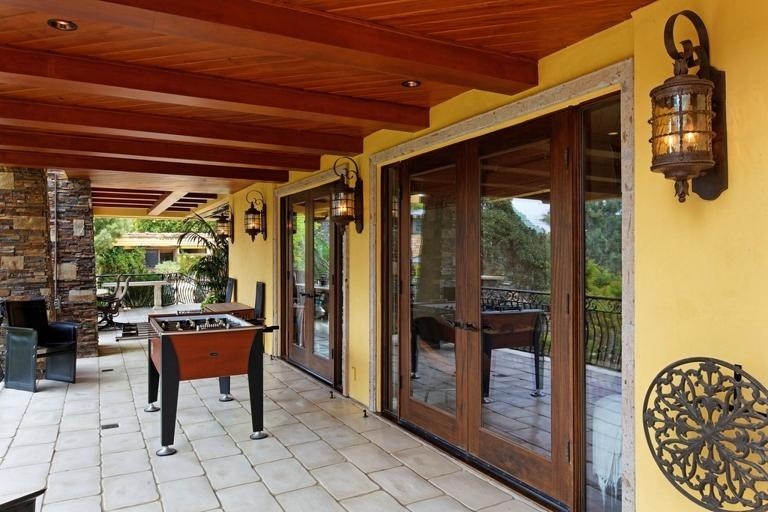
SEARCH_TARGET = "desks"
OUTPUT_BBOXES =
[203,302,256,321]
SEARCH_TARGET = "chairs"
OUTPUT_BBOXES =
[96,273,131,331]
[3,297,79,393]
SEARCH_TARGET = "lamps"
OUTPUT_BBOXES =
[329,156,364,237]
[645,9,729,205]
[242,189,267,243]
[216,202,234,250]
